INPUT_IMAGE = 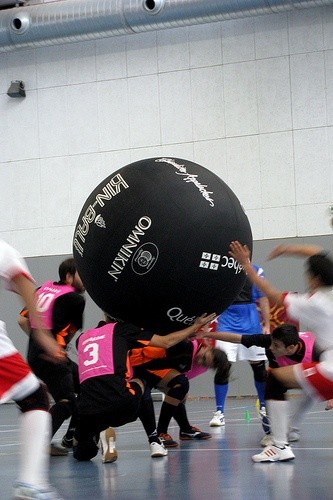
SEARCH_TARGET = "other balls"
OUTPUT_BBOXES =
[72,156,255,333]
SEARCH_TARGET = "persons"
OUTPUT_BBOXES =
[17,240,333,464]
[0,240,69,500]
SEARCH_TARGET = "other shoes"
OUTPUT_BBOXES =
[99,429,117,464]
[48,442,68,456]
[149,441,167,458]
[261,426,300,446]
[12,480,62,500]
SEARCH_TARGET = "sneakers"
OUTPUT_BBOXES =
[159,433,178,447]
[179,426,211,440]
[250,444,295,463]
[208,411,225,426]
